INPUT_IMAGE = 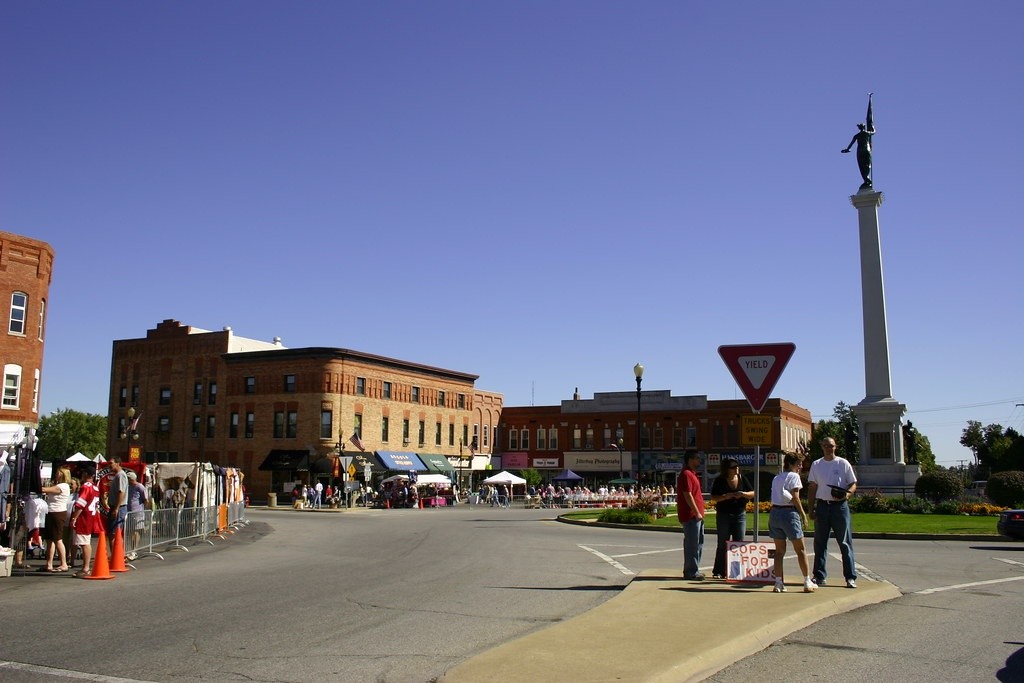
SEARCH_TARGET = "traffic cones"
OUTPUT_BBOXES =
[83,531,115,579]
[386,499,390,509]
[109,527,130,572]
[420,499,423,509]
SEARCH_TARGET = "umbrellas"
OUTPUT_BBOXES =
[608,479,638,484]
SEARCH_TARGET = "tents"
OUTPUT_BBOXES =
[482,470,526,495]
[551,469,583,481]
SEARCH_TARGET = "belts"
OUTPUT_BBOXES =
[772,504,793,509]
[120,505,126,507]
[818,498,841,504]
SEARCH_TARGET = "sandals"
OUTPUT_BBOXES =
[72,569,91,578]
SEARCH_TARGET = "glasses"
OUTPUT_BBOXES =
[820,444,836,449]
[726,464,739,469]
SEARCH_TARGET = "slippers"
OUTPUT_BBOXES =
[712,574,721,579]
[50,566,68,573]
[15,564,29,568]
[35,566,52,572]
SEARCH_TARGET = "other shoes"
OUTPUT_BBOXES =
[128,552,138,560]
[804,580,818,592]
[811,577,827,584]
[847,578,857,588]
[773,581,788,592]
[686,573,706,581]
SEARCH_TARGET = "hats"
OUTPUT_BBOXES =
[826,484,852,499]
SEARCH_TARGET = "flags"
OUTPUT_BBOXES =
[349,433,365,452]
[130,414,142,430]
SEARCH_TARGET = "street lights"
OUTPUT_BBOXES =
[619,438,623,479]
[459,437,463,495]
[634,362,644,491]
[121,406,139,456]
[334,427,345,456]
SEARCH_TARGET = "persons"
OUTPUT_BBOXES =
[70,466,99,577]
[768,452,818,592]
[808,437,858,587]
[108,457,128,553]
[126,472,149,560]
[292,480,338,509]
[478,485,511,508]
[530,483,674,508]
[677,450,706,582]
[361,484,373,504]
[15,529,44,568]
[846,124,875,185]
[711,458,754,579]
[36,467,81,573]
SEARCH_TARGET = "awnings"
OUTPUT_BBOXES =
[258,449,309,471]
[341,450,455,473]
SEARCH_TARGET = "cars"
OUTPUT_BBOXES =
[971,481,988,497]
[997,510,1024,539]
